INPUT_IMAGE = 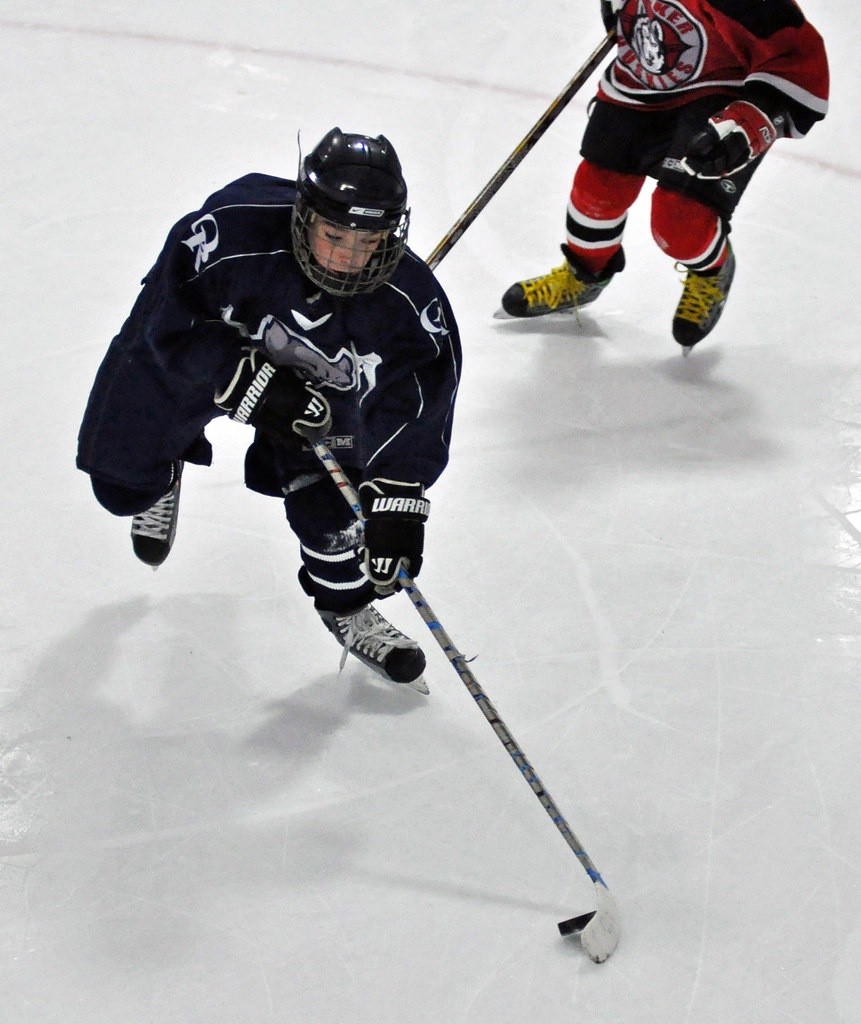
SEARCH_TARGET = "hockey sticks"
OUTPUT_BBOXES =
[309,436,620,965]
[424,29,617,269]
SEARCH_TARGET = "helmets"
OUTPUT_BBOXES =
[292,126,410,295]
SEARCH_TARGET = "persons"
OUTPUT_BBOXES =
[76,123,462,694]
[495,0,829,357]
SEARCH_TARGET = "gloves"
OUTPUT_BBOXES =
[357,475,428,601]
[600,0,627,33]
[684,98,778,180]
[214,352,332,446]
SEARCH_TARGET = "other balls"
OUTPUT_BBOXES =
[558,909,595,937]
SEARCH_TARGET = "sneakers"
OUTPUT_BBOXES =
[131,458,185,571]
[672,236,731,358]
[313,599,430,695]
[493,242,624,319]
